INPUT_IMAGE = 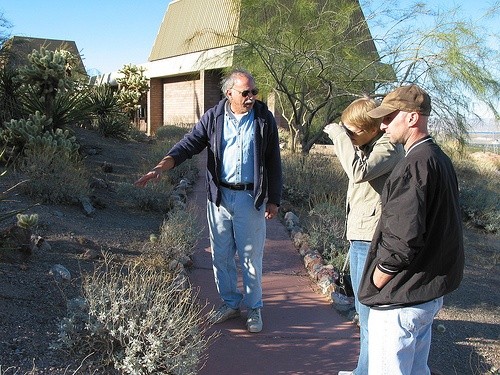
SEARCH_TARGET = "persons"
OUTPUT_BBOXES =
[355,85,464,375]
[324,97,405,375]
[135,70,282,333]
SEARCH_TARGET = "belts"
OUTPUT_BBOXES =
[219,182,254,190]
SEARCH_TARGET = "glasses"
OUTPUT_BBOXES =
[345,128,363,137]
[232,88,259,97]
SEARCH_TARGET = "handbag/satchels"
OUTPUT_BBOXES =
[333,247,355,298]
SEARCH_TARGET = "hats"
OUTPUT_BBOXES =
[367,88,432,118]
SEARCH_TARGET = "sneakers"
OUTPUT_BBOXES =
[207,305,241,324]
[245,307,263,333]
[338,371,355,375]
